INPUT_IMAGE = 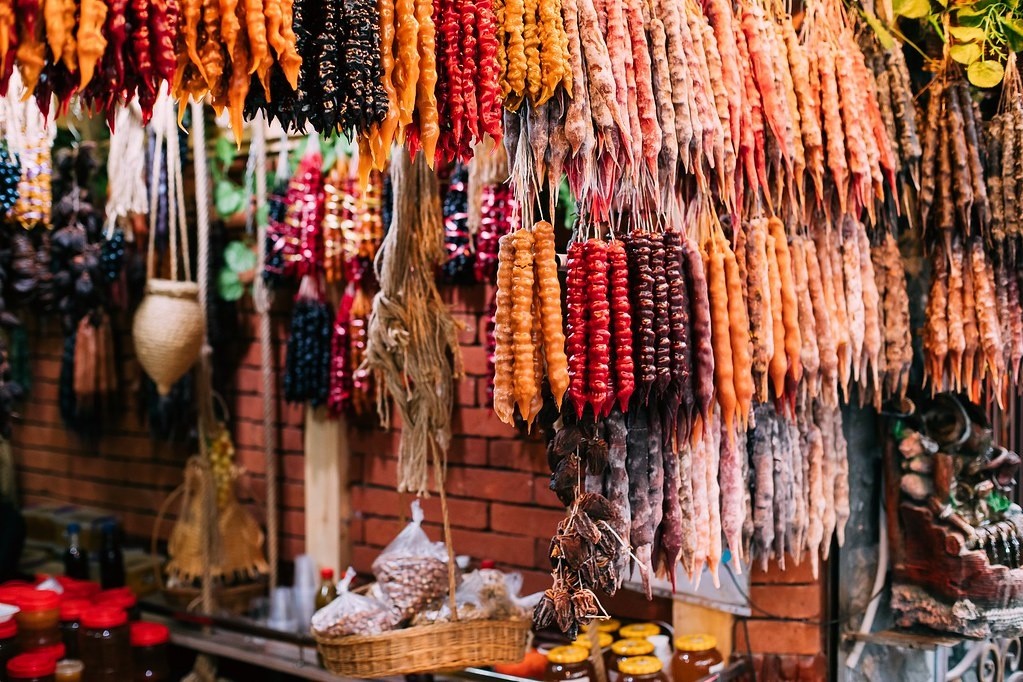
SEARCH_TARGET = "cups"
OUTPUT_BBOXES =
[266,554,314,633]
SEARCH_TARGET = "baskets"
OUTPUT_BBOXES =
[309,581,533,678]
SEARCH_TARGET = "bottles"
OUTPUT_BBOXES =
[314,570,356,670]
[0,517,173,682]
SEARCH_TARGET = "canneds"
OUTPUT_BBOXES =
[546,617,726,682]
